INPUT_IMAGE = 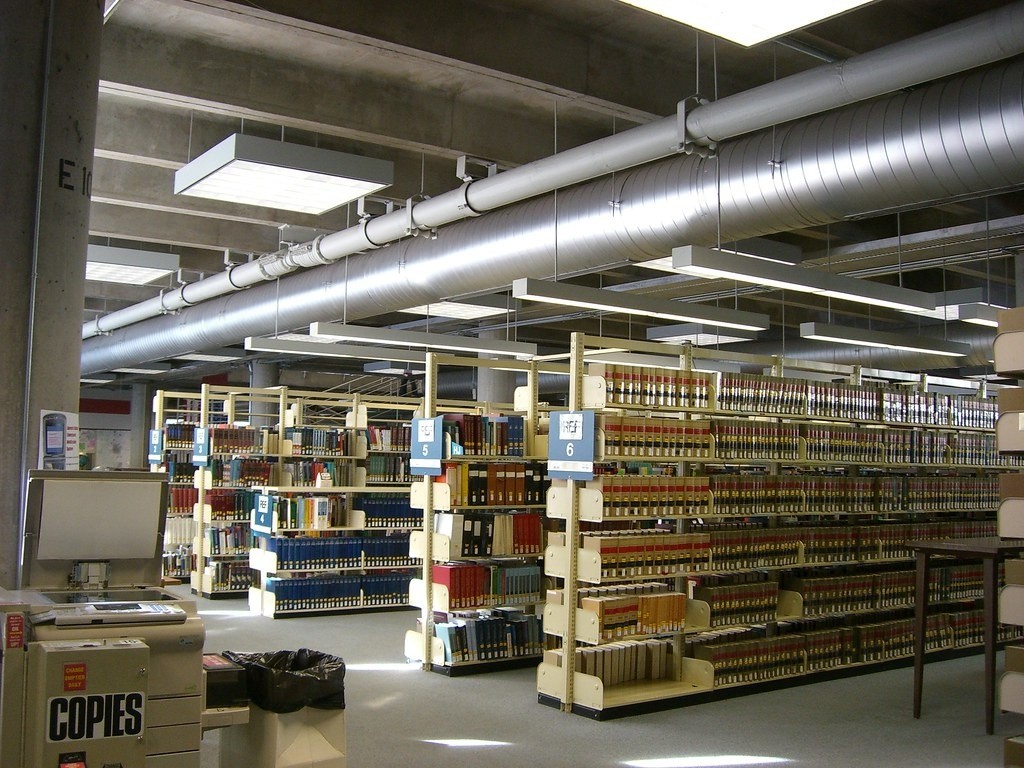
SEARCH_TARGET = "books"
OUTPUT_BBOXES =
[544,361,1024,689]
[416,411,564,662]
[161,419,423,611]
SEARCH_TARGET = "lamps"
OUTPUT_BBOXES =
[85,119,1011,390]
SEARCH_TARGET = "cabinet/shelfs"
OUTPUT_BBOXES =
[152,331,1023,768]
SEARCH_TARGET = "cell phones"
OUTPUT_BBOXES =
[42,413,67,470]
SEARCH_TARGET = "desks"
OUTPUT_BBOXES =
[901,537,1024,735]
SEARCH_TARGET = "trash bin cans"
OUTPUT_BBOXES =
[219,647,346,768]
